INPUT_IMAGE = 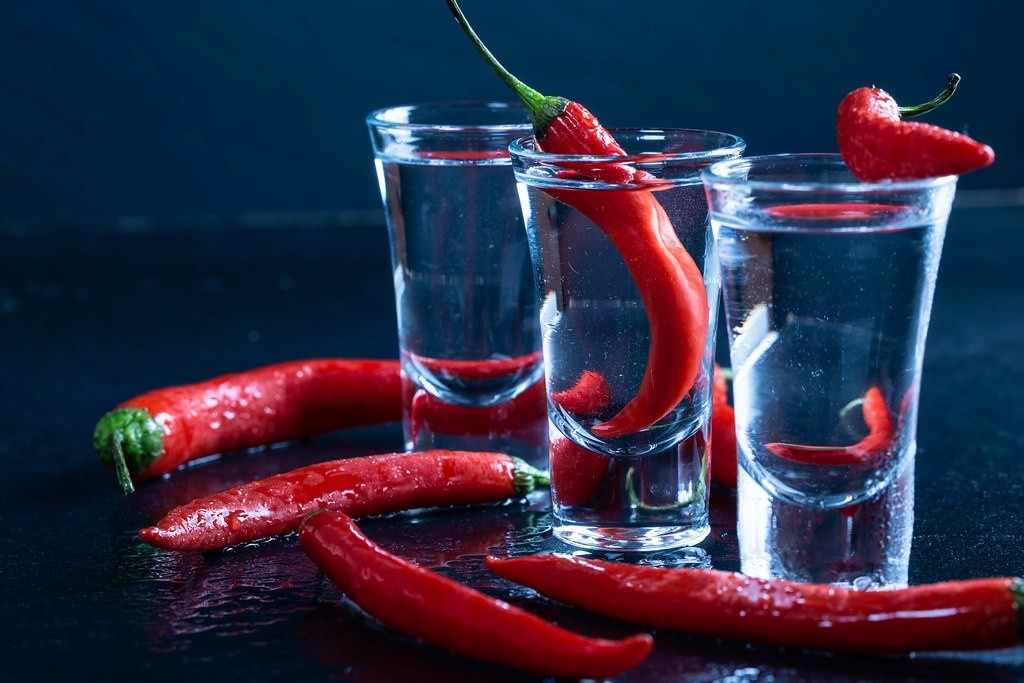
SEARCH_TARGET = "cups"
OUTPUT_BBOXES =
[367,103,549,479]
[698,153,961,589]
[507,125,748,553]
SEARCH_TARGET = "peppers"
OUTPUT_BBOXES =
[446,0,707,436]
[95,357,1024,676]
[839,72,994,183]
